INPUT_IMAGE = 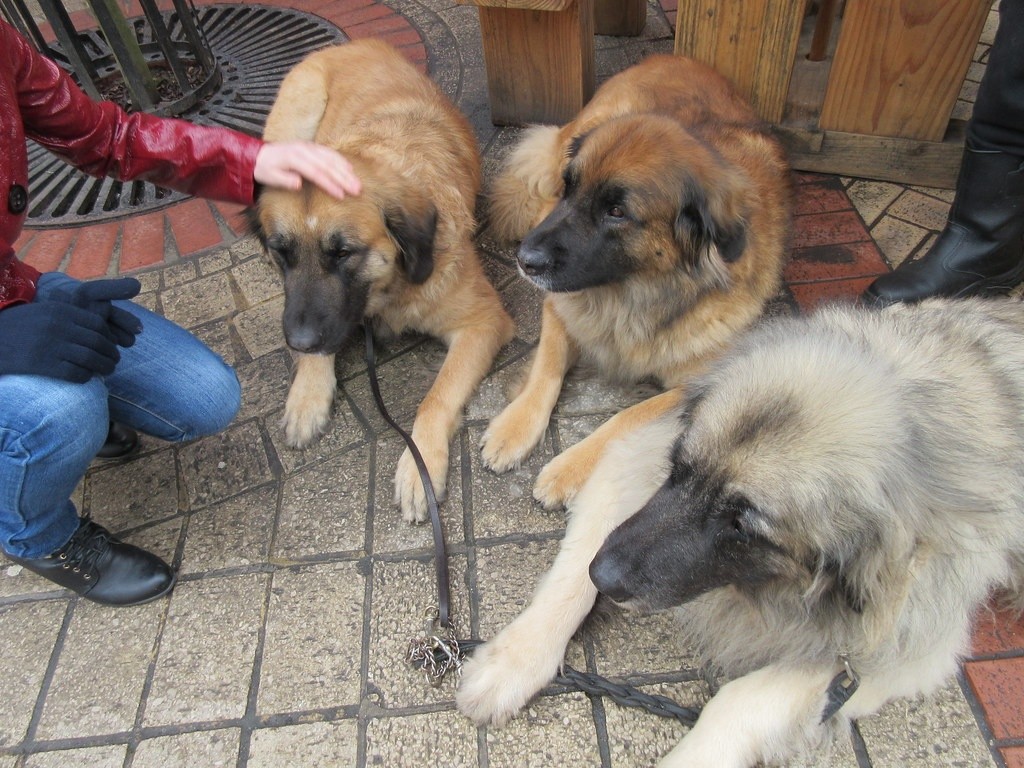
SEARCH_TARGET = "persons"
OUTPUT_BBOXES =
[0,15,362,607]
[863,0,1024,306]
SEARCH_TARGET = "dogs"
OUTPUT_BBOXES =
[451,291,1024,766]
[245,36,523,524]
[479,53,790,512]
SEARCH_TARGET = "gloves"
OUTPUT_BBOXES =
[0,277,143,384]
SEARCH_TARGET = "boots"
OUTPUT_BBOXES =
[863,143,1024,309]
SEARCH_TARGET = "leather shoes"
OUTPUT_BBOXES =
[95,421,137,460]
[0,517,177,608]
